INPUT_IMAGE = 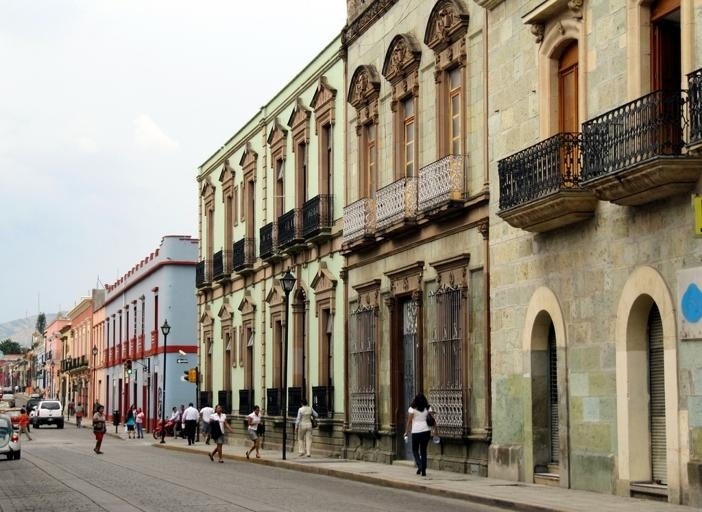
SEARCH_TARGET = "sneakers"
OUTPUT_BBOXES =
[246,451,249,459]
[209,453,214,460]
[421,470,426,475]
[219,459,224,463]
[417,464,422,474]
[256,454,260,458]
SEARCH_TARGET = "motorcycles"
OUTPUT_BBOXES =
[153,413,187,439]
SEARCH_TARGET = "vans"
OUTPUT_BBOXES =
[33,399,64,429]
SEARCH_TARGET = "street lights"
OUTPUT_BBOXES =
[51,360,55,400]
[277,265,298,460]
[91,344,98,418]
[160,318,171,443]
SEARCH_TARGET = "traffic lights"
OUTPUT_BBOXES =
[182,368,197,383]
[127,357,133,375]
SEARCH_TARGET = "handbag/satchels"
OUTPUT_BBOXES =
[257,422,265,434]
[210,421,222,440]
[92,420,106,433]
[311,418,317,428]
[426,413,435,426]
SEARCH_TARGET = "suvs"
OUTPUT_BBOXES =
[0,412,22,461]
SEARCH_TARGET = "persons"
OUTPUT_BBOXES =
[296,400,319,457]
[246,406,262,459]
[404,392,437,476]
[17,390,215,454]
[209,404,232,462]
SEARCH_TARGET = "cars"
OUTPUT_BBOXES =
[22,398,43,424]
[4,407,30,432]
[0,400,10,415]
[2,393,16,408]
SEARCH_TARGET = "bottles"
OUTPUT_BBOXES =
[404,434,409,444]
